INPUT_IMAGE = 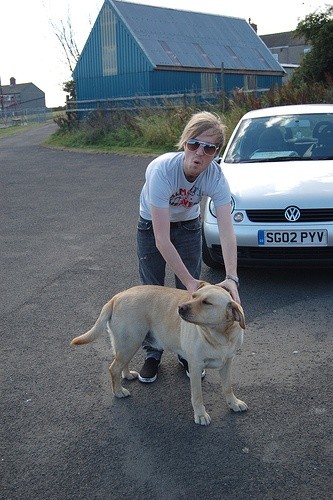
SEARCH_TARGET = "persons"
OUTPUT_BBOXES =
[136,109,242,383]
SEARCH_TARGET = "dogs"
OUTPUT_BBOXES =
[72,281,248,426]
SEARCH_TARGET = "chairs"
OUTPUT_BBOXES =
[308,121,333,157]
[250,123,297,159]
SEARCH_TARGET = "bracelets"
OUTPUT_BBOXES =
[225,274,239,286]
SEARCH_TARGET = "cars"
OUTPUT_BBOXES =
[201,104,333,271]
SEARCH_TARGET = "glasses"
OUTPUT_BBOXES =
[186,139,221,156]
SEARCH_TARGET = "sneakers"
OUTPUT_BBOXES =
[178,355,206,379]
[138,354,162,383]
